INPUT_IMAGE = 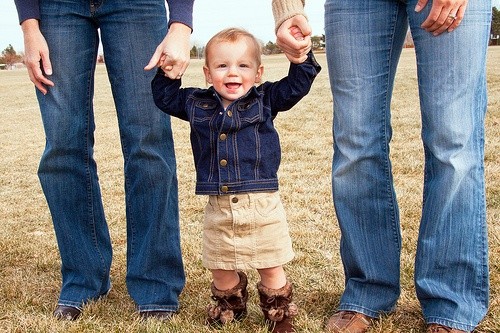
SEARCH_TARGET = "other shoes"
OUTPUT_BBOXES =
[425,322,467,333]
[51,304,79,324]
[326,309,375,333]
[139,308,176,319]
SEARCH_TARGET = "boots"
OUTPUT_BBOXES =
[205,271,251,329]
[257,280,297,332]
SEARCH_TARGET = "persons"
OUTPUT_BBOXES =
[152,25,322,333]
[270,0,494,332]
[13,0,194,318]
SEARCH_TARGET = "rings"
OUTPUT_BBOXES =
[178,72,184,76]
[448,13,457,20]
